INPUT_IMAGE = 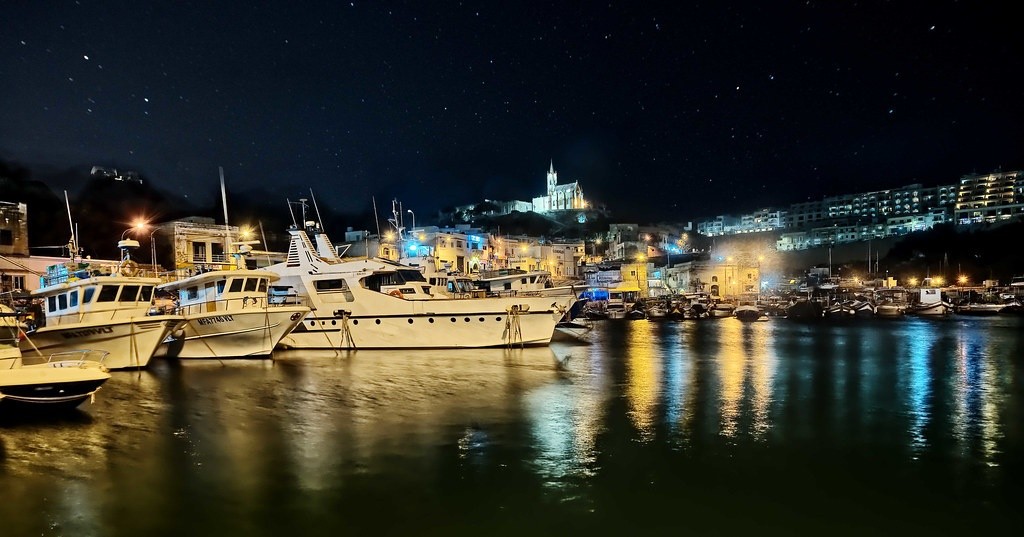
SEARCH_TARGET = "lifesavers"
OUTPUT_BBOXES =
[390,290,403,299]
[121,260,139,277]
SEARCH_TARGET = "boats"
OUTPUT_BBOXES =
[0,171,1024,411]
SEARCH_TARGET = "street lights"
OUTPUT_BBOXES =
[407,209,415,230]
[121,220,147,261]
